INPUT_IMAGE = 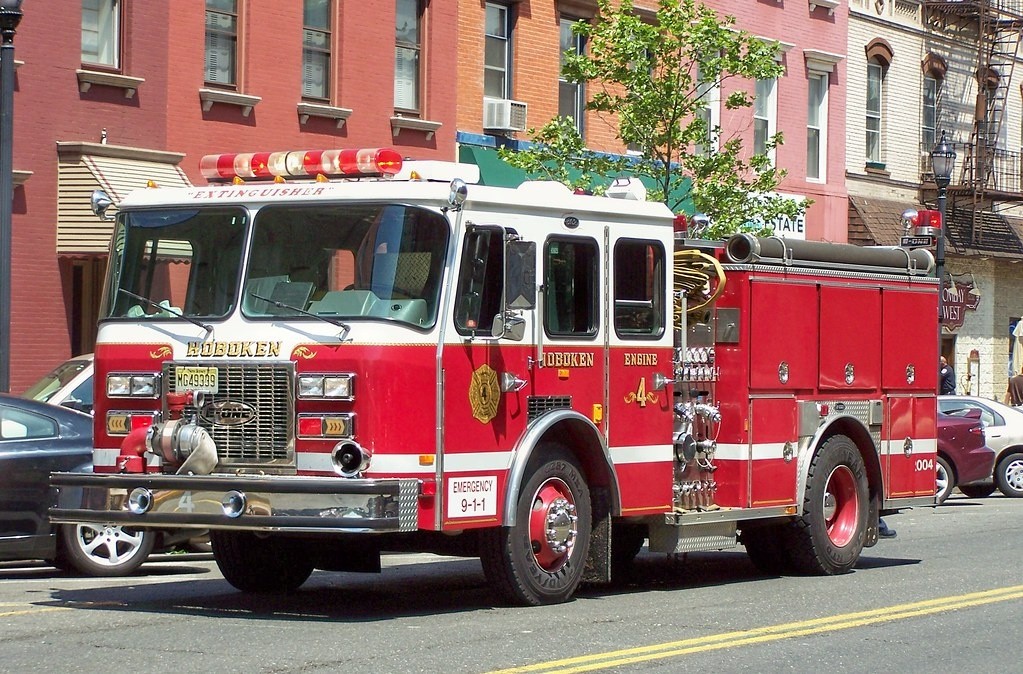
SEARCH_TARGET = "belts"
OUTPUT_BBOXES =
[1012,404,1021,406]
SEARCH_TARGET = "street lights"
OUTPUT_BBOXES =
[928,130,957,394]
[1,0,26,394]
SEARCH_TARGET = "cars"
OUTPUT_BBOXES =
[0,352,156,576]
[933,394,1023,505]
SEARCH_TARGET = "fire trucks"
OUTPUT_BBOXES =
[46,146,940,608]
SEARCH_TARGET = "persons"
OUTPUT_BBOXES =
[1003,366,1023,410]
[940,356,956,395]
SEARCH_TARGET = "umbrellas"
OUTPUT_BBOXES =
[1012,316,1023,376]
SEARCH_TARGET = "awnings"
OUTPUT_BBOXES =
[57,153,195,265]
[458,144,702,226]
